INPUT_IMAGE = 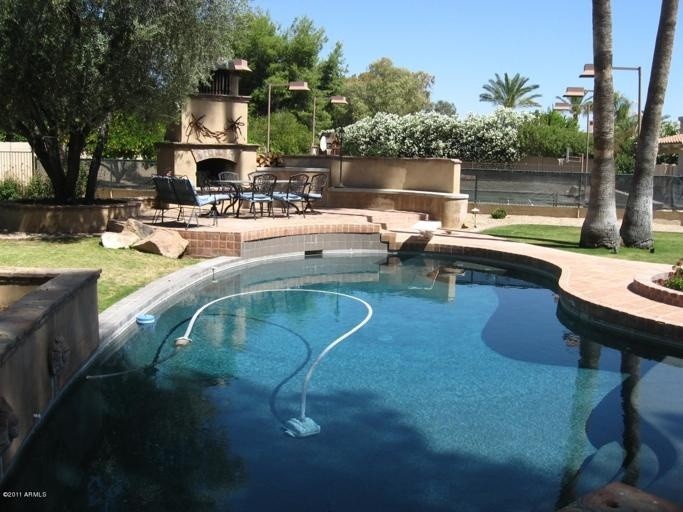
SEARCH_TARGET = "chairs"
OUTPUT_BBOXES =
[218,170,327,216]
[151,174,217,227]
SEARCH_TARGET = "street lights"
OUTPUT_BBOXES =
[553,62,642,208]
[312,96,347,145]
[267,81,311,154]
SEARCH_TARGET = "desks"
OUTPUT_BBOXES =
[208,179,312,214]
